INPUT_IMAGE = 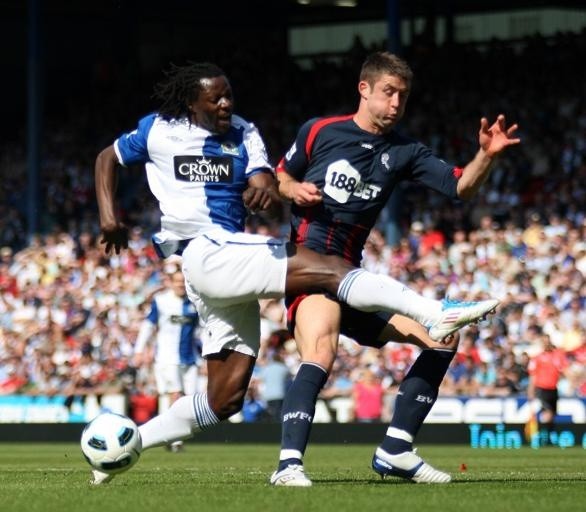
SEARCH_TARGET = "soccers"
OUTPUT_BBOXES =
[81,411,142,475]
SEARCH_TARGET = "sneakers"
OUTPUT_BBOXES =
[427,297,503,344]
[91,467,111,485]
[371,442,454,485]
[268,464,313,489]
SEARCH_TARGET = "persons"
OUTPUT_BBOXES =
[0,21,585,425]
[266,50,523,488]
[90,60,502,485]
